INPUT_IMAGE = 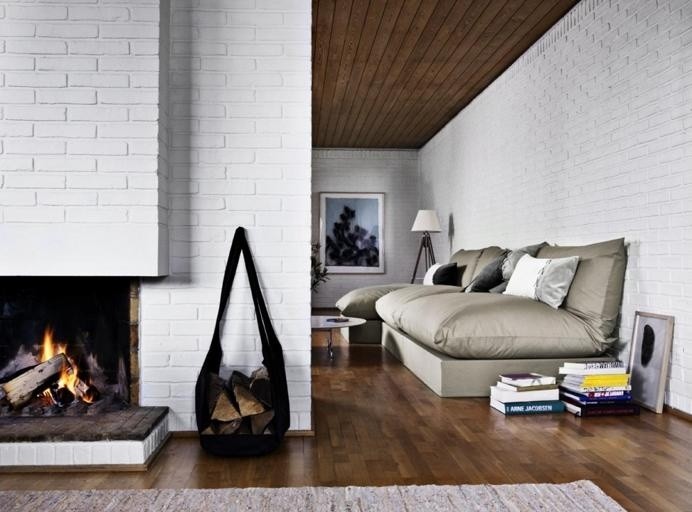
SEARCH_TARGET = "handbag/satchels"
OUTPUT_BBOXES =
[193,224,290,458]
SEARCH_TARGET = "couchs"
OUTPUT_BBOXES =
[335,238,626,396]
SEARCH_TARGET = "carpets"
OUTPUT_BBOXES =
[0,479,627,512]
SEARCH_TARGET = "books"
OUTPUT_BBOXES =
[490,360,641,417]
[327,318,349,322]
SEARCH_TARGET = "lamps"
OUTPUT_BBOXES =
[409,210,443,284]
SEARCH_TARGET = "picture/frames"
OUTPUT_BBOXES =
[625,311,675,413]
[318,191,386,274]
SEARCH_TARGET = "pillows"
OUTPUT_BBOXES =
[422,252,582,310]
